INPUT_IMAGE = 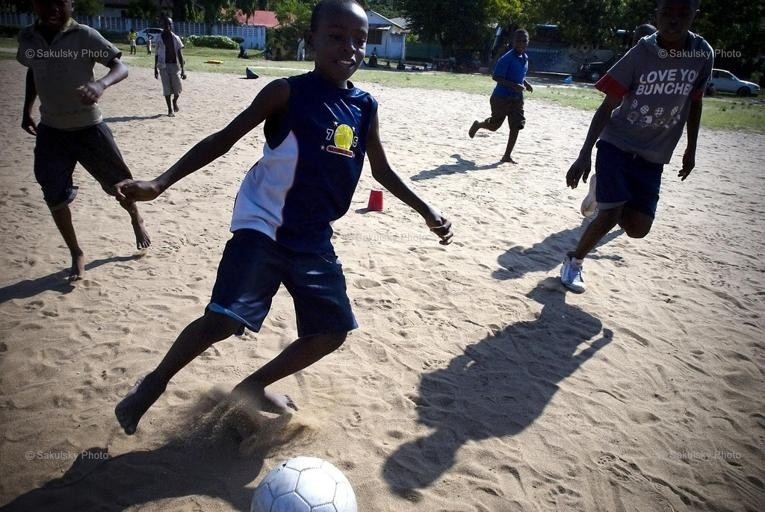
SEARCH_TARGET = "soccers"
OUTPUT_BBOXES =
[250,456,357,512]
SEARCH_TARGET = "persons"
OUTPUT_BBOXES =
[126,28,138,55]
[145,28,154,55]
[154,16,187,117]
[14,1,153,281]
[297,34,306,61]
[631,22,658,47]
[468,28,535,165]
[471,47,481,69]
[111,1,455,438]
[559,0,717,296]
[238,44,249,59]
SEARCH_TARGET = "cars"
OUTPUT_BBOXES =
[703,68,761,97]
[131,28,165,45]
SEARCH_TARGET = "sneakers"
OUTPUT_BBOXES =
[581,174,598,217]
[560,252,586,293]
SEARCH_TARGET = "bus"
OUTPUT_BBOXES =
[526,23,630,76]
